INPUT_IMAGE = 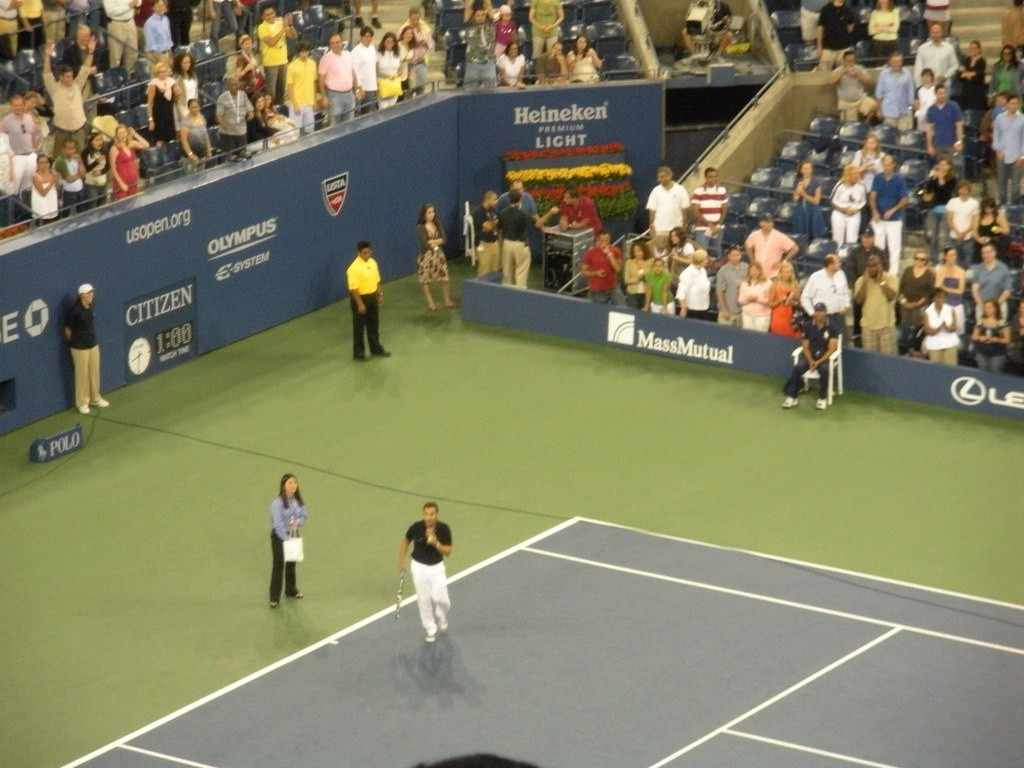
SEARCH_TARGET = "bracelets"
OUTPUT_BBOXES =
[78,160,83,166]
[716,223,722,229]
[243,68,248,73]
[880,281,886,287]
[679,257,682,265]
[899,299,908,305]
[608,252,614,257]
[147,115,153,121]
[649,224,654,227]
[187,151,192,158]
[435,541,440,549]
[378,292,383,297]
[708,220,712,227]
[289,23,293,27]
[87,51,95,56]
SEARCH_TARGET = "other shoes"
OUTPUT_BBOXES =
[355,357,368,361]
[439,613,448,630]
[426,634,436,642]
[372,352,391,356]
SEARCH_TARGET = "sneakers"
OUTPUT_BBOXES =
[76,404,90,414]
[288,592,303,598]
[816,398,827,409]
[90,398,109,407]
[782,396,798,408]
[270,601,278,607]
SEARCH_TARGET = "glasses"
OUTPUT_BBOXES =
[21,124,26,133]
[915,257,926,261]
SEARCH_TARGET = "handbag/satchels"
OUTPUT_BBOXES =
[378,76,403,99]
[244,70,269,95]
[283,525,304,562]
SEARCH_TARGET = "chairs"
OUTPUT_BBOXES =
[0,1,1024,371]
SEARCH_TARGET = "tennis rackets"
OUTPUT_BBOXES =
[395,576,404,620]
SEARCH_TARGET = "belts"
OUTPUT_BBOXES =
[17,152,32,155]
[111,18,132,23]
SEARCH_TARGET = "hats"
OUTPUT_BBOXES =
[861,228,873,238]
[814,302,826,311]
[500,5,512,15]
[78,283,94,297]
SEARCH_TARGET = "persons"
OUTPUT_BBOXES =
[398,501,453,642]
[267,472,308,608]
[0,0,1024,416]
